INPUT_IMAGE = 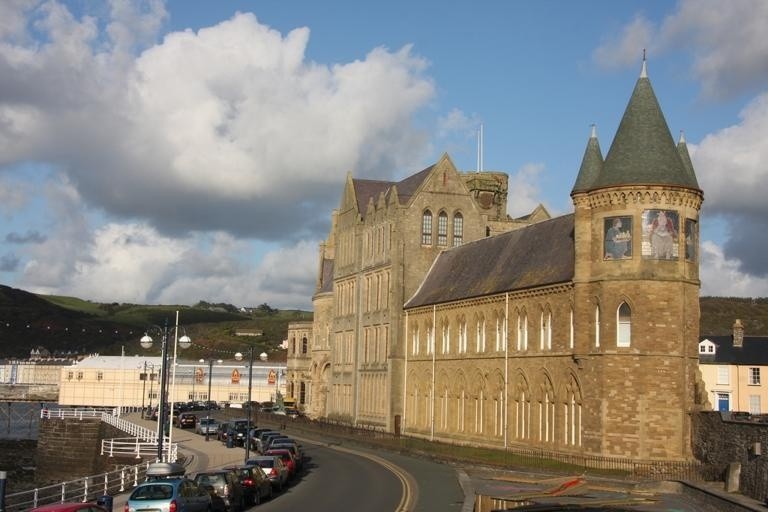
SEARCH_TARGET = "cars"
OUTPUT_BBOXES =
[20,502,109,512]
[122,395,306,512]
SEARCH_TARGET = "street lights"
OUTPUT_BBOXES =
[136,321,191,462]
[234,348,269,463]
[198,357,223,441]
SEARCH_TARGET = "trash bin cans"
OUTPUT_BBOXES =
[226,432,234,448]
[97,496,113,512]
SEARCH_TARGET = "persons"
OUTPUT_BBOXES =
[605,218,628,258]
[649,212,678,260]
